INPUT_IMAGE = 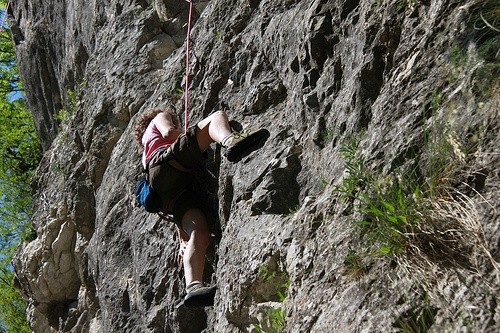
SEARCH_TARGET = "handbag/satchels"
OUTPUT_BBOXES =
[133,179,161,212]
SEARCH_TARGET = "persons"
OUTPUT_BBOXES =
[133,106,270,306]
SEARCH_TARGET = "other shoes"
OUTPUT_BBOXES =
[184,280,218,306]
[220,128,270,162]
